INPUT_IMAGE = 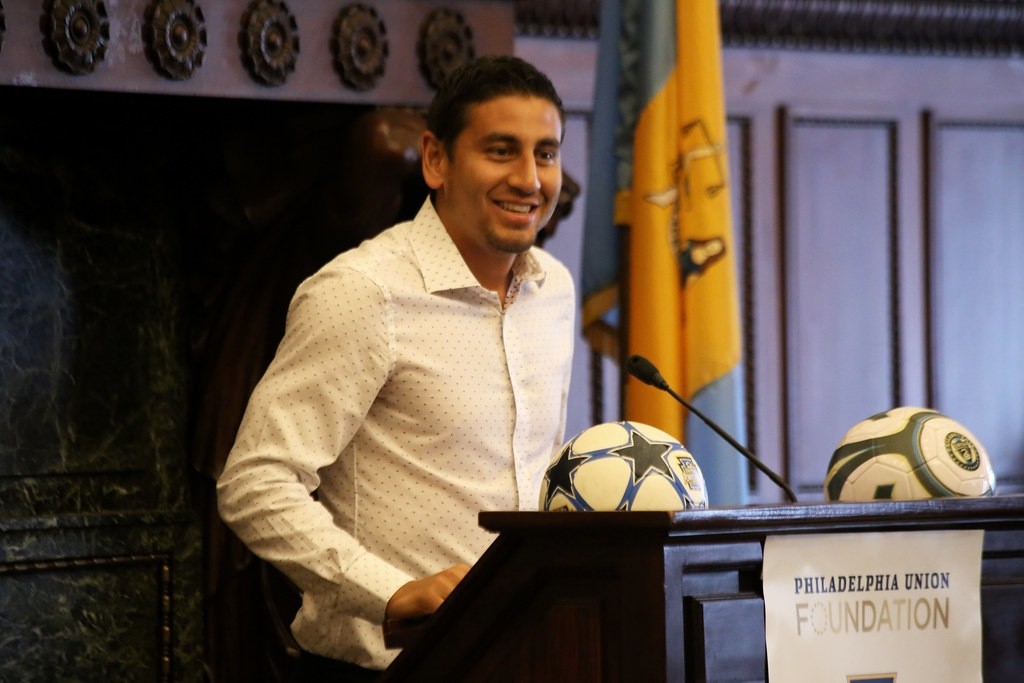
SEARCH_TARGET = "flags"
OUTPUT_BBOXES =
[578,0,751,509]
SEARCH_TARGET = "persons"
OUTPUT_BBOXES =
[216,56,577,683]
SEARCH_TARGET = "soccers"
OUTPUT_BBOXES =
[824,405,998,504]
[537,420,710,513]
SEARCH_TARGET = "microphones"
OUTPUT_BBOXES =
[622,354,799,504]
